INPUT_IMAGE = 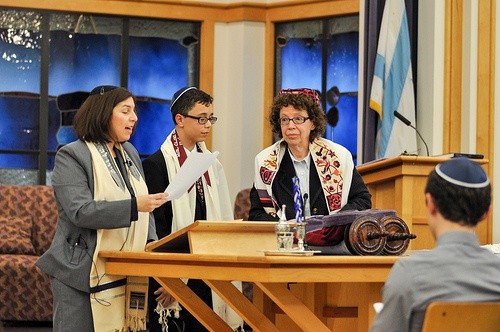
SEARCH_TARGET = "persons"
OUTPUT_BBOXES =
[34,85,166,332]
[247,88,373,222]
[141,85,225,332]
[373,156,500,332]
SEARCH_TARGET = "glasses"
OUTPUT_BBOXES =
[325,86,340,128]
[183,115,218,125]
[278,115,311,125]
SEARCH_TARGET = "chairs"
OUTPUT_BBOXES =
[421,301,500,332]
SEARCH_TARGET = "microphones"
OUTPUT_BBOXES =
[129,161,132,166]
[394,111,429,156]
[302,193,309,222]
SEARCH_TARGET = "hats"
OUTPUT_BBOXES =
[435,158,489,188]
[170,87,197,112]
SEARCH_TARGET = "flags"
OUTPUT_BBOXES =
[370,0,417,160]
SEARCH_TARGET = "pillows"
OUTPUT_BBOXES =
[0,213,37,257]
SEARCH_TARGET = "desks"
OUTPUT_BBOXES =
[99,249,406,332]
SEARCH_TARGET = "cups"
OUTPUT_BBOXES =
[275,225,290,250]
[278,231,293,251]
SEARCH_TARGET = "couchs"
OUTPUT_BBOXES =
[0,183,59,332]
